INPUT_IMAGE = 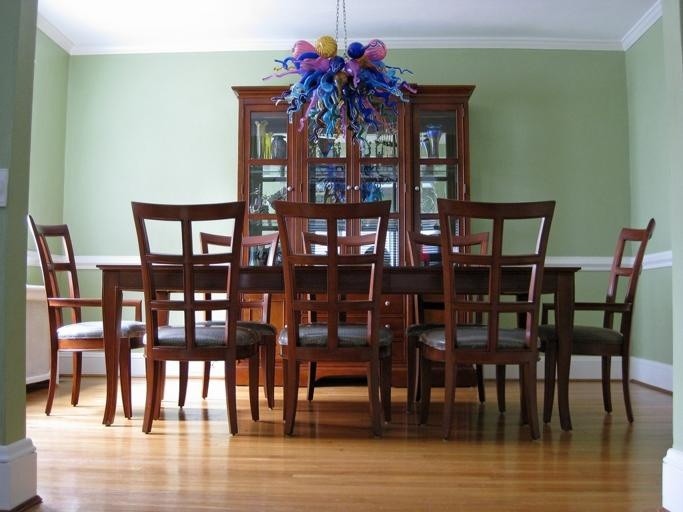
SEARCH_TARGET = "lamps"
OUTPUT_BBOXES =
[255,1,425,160]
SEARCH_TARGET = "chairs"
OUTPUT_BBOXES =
[406,230,490,414]
[494,218,657,431]
[26,215,146,424]
[130,201,279,435]
[175,232,277,409]
[300,232,376,402]
[406,197,561,441]
[275,199,394,437]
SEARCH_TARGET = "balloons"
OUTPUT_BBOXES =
[292,35,386,88]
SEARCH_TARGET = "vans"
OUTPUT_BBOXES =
[258,182,440,267]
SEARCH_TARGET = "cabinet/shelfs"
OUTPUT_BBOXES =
[221,80,487,390]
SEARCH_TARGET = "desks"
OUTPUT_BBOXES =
[96,264,582,436]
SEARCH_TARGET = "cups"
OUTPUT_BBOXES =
[427,123,442,157]
[254,120,271,160]
